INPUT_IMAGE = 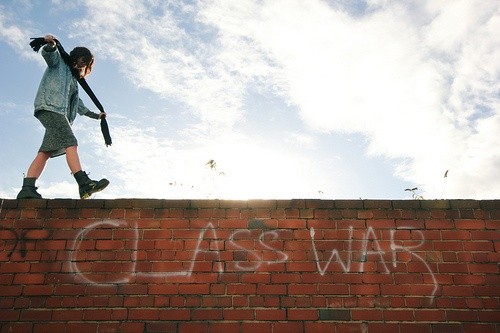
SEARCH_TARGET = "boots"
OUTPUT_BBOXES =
[74,170,109,198]
[18,177,41,198]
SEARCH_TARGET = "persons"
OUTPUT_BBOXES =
[16,35,110,200]
